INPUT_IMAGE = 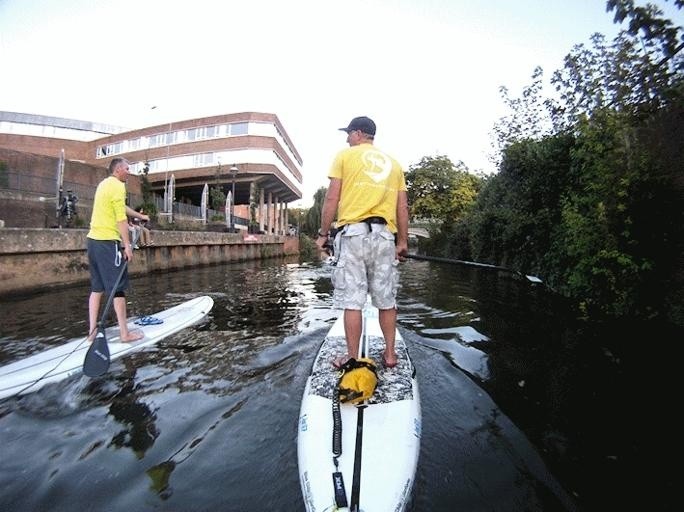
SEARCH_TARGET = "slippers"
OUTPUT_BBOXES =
[134,315,163,325]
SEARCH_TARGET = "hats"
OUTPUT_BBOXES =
[338,117,376,135]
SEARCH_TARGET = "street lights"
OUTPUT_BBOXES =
[229,163,238,232]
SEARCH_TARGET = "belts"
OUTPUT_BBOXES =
[338,216,387,231]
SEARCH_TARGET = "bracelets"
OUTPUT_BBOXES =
[318,232,329,237]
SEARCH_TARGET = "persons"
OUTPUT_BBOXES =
[86,156,152,344]
[313,116,410,368]
[126,201,141,249]
[132,206,155,248]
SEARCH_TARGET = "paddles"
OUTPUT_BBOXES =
[322,242,543,283]
[82,219,148,378]
